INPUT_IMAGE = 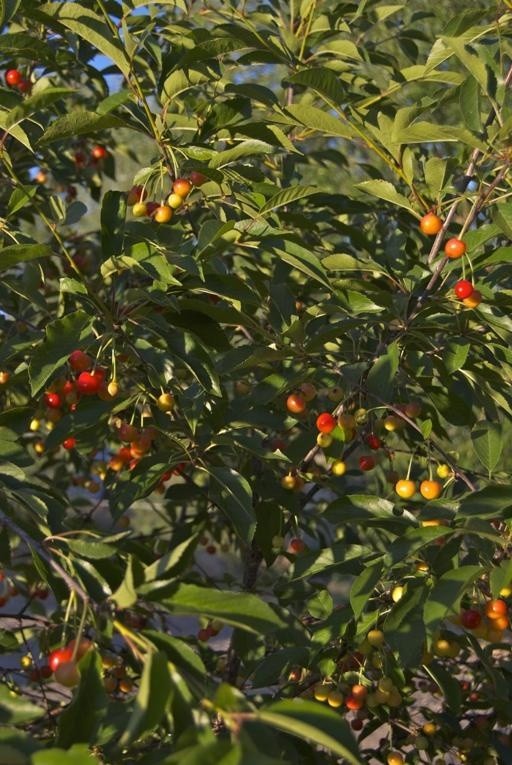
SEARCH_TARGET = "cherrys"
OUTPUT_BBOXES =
[1,67,511,764]
